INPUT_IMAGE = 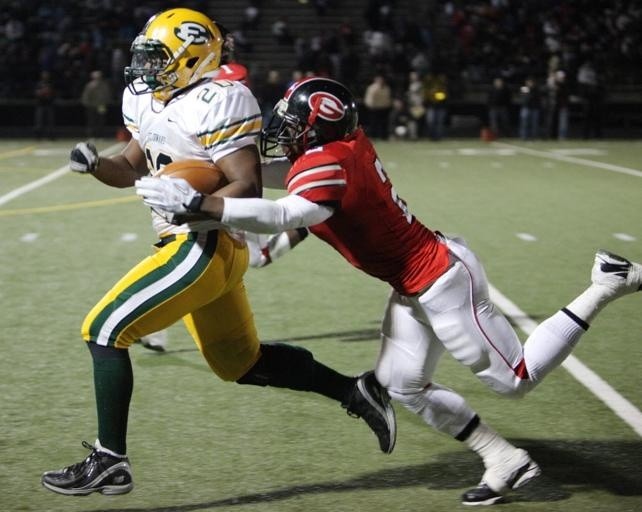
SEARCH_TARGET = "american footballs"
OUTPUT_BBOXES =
[154,161,228,194]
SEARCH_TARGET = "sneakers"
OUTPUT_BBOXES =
[461,448,542,507]
[343,370,396,454]
[41,442,134,496]
[592,247,641,295]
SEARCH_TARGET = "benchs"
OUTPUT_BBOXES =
[188,0,642,107]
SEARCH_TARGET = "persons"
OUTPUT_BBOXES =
[0,0,166,143]
[229,0,642,143]
[133,73,642,508]
[40,5,399,498]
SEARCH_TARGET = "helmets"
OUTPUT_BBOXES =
[257,77,359,164]
[123,9,224,103]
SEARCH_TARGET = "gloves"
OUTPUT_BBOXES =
[136,173,205,227]
[69,141,102,174]
